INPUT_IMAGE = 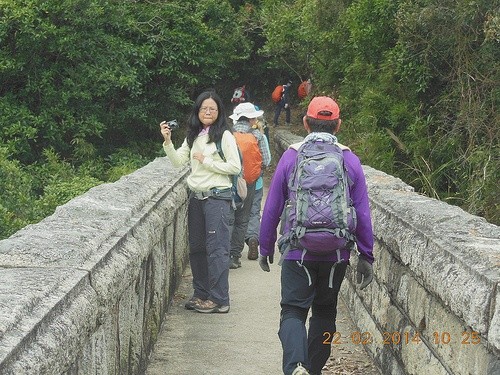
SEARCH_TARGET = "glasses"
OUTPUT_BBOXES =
[200,107,216,114]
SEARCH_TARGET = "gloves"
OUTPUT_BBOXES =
[356,256,373,290]
[259,255,273,272]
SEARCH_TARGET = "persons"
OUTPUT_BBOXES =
[259,96,374,375]
[298,76,313,99]
[272,80,293,124]
[231,83,248,106]
[159,90,247,313]
[226,101,271,269]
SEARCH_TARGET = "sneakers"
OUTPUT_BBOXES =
[185,298,203,308]
[193,299,229,313]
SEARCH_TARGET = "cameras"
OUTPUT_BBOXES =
[166,120,179,132]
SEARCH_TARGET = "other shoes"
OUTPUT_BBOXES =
[286,123,291,125]
[273,123,278,128]
[229,255,241,269]
[248,238,259,260]
[292,362,309,375]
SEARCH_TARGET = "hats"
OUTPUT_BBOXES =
[307,96,339,120]
[227,102,264,121]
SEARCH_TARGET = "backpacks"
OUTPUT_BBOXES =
[289,137,355,254]
[230,126,261,184]
[297,81,308,99]
[271,85,285,103]
[233,88,245,103]
